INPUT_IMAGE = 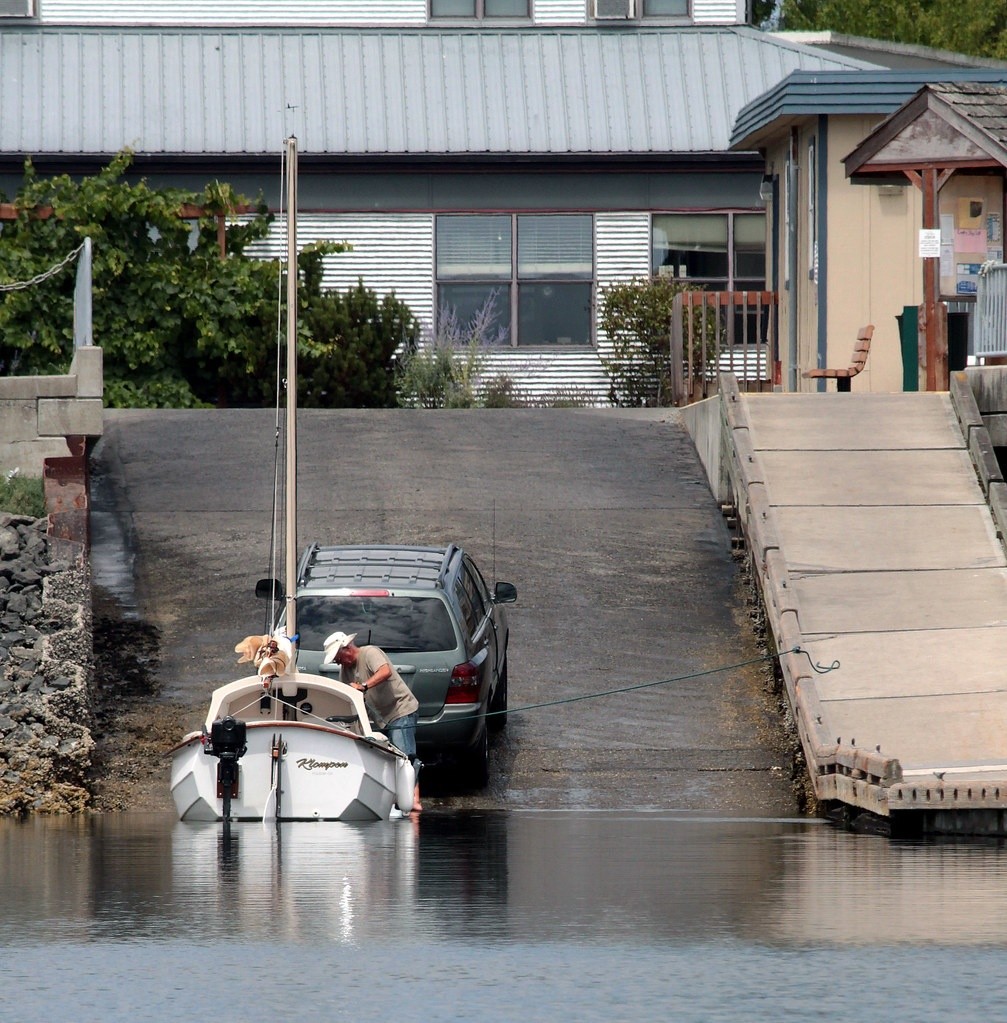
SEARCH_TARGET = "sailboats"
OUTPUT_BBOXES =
[164,105,422,824]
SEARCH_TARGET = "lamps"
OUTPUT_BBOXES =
[758,174,773,201]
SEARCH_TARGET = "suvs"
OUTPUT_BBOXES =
[255,542,518,794]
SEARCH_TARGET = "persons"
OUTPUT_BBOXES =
[323,631,423,811]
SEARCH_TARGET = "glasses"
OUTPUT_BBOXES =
[333,655,341,662]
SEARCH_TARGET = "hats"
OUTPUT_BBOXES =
[322,630,357,664]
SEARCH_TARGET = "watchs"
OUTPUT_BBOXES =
[360,682,368,690]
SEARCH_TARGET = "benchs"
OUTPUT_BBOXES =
[802,324,876,392]
